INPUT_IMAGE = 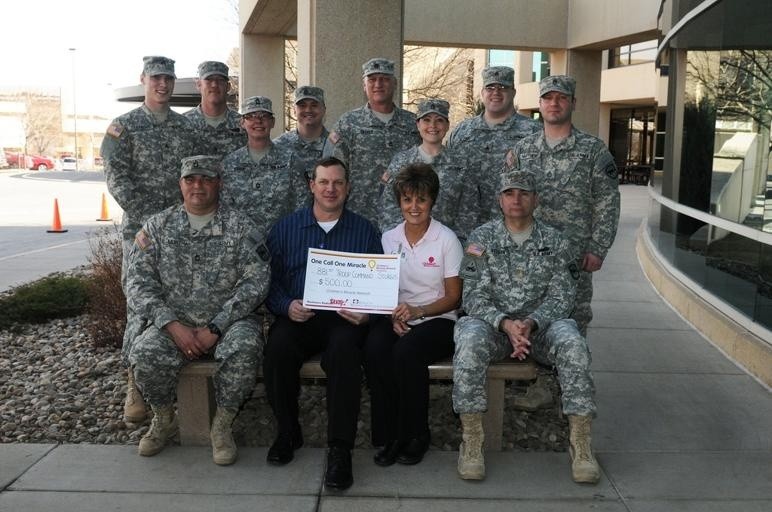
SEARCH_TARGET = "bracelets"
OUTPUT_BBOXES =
[419,306,427,320]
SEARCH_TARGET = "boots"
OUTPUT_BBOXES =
[125,368,147,422]
[138,401,178,457]
[457,413,486,480]
[210,406,238,465]
[568,413,602,483]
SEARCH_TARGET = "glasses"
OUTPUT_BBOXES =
[184,176,213,185]
[485,85,509,91]
[243,113,273,120]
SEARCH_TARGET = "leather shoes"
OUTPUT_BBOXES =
[268,425,304,464]
[374,439,403,466]
[325,446,353,492]
[395,439,428,465]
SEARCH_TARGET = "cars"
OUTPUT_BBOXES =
[6,152,55,171]
[63,157,78,171]
[59,154,71,162]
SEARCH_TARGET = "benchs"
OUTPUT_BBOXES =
[176,359,539,452]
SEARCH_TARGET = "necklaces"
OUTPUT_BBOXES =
[406,230,423,247]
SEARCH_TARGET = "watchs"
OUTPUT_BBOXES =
[208,323,222,337]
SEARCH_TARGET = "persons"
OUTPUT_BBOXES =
[453,169,603,483]
[490,75,620,338]
[100,55,203,422]
[263,156,384,493]
[271,85,330,177]
[322,58,423,234]
[125,155,271,466]
[445,65,543,227]
[380,99,482,246]
[181,61,248,165]
[217,96,311,242]
[371,164,463,467]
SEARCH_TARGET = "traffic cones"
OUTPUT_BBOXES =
[46,197,67,232]
[96,193,112,221]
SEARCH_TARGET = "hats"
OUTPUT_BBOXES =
[540,75,575,97]
[241,96,273,116]
[198,61,229,81]
[417,100,449,121]
[143,57,177,79]
[294,86,323,105]
[482,67,514,88]
[499,171,537,193]
[361,58,395,79]
[180,155,224,181]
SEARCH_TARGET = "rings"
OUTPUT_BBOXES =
[401,315,404,320]
[187,351,191,354]
